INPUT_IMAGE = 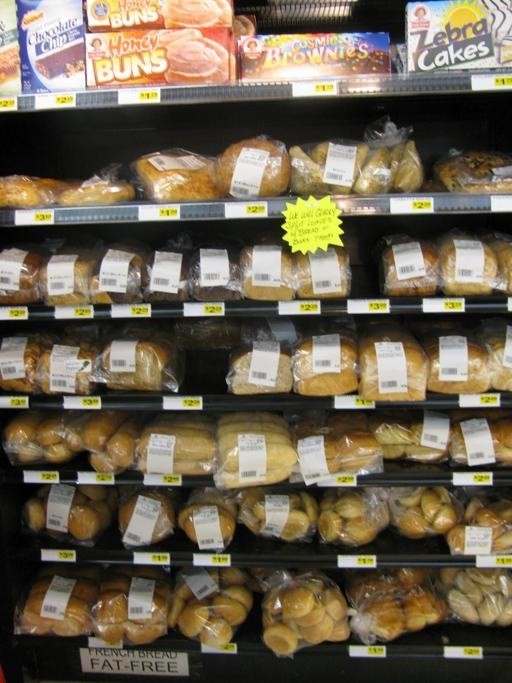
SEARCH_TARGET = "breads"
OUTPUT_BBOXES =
[0,230,512,308]
[18,562,512,658]
[1,408,512,556]
[153,36,233,86]
[0,137,512,208]
[0,316,512,402]
[161,0,233,27]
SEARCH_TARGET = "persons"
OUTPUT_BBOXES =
[91,38,104,58]
[247,40,260,58]
[411,6,429,27]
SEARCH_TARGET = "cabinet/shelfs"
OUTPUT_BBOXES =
[0,66,512,683]
